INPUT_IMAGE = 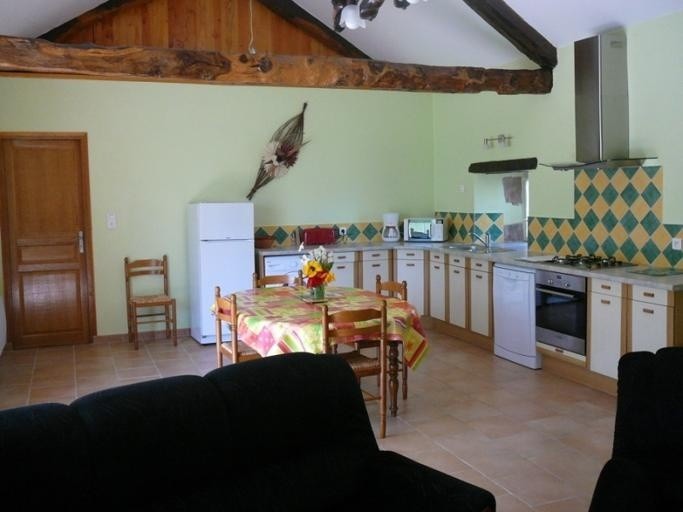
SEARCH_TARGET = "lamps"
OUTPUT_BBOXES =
[327,0,425,35]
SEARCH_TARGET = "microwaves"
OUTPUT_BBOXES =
[403,217,449,243]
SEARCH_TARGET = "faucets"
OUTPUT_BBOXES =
[465,229,494,250]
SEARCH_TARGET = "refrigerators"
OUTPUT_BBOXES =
[187,202,257,346]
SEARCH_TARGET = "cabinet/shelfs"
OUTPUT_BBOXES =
[320,249,356,289]
[446,258,470,329]
[627,282,683,355]
[392,250,427,319]
[254,244,310,289]
[585,279,626,377]
[358,250,392,294]
[428,252,449,322]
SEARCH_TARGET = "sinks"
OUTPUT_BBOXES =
[443,241,514,254]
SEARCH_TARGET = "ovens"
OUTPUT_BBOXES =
[534,269,589,356]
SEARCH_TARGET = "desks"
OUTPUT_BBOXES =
[208,286,427,420]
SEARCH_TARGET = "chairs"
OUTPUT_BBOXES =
[215,286,263,368]
[351,274,406,401]
[123,253,178,351]
[251,275,292,289]
[322,300,387,438]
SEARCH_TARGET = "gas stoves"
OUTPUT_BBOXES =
[535,253,639,272]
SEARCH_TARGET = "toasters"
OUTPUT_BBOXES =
[300,228,336,246]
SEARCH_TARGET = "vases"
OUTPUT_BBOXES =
[310,283,324,300]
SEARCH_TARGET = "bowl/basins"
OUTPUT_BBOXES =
[255,236,276,248]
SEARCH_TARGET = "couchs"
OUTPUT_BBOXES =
[0,352,496,512]
[589,342,683,512]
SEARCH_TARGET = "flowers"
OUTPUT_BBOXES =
[298,240,337,288]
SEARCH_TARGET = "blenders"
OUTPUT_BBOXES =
[381,212,400,242]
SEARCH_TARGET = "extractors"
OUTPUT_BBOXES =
[539,25,659,172]
